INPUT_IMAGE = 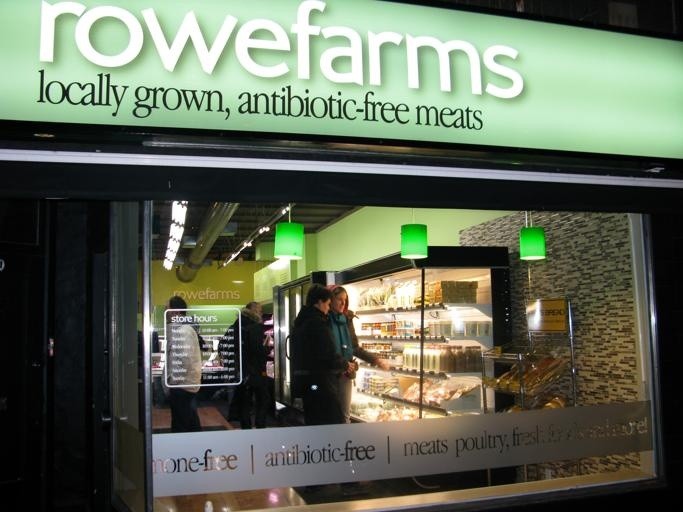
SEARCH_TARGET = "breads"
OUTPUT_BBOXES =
[491,355,569,414]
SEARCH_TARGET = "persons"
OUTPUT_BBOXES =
[164,295,202,431]
[290,283,354,424]
[233,302,271,428]
[326,285,389,423]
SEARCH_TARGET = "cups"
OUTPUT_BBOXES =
[428,321,490,337]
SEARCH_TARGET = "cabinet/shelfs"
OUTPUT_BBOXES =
[156,333,225,404]
[261,303,276,380]
[326,247,512,487]
[272,271,324,426]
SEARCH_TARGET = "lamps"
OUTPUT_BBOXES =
[162,200,188,270]
[273,203,304,259]
[518,211,546,262]
[216,203,295,269]
[400,208,429,258]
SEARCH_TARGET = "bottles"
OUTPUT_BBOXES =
[403,344,482,372]
